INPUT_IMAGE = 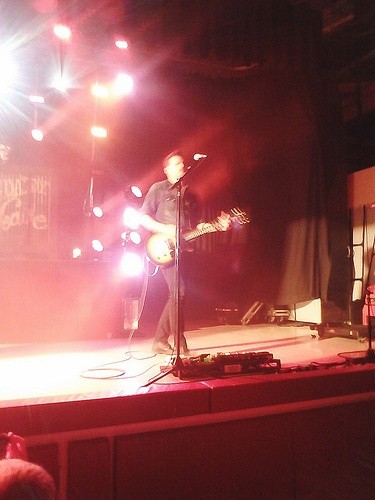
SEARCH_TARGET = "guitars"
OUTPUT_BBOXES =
[145,207,250,269]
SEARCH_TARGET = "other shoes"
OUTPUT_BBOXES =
[174,336,188,354]
[152,341,174,355]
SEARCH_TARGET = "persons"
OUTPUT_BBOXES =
[136,148,232,355]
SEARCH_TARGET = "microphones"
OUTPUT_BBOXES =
[193,153,207,160]
